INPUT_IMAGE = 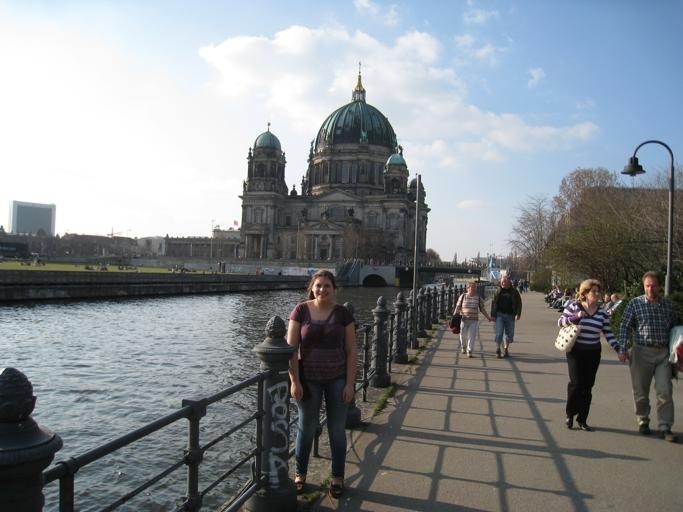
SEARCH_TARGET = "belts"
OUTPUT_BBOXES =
[642,343,663,347]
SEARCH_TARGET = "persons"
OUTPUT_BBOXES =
[286,270,356,501]
[489,274,521,360]
[617,270,680,445]
[451,281,490,359]
[557,280,628,429]
[503,274,622,317]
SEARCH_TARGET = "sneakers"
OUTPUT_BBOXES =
[658,429,678,441]
[329,478,344,498]
[294,478,305,494]
[500,351,507,358]
[467,350,472,357]
[460,348,465,353]
[495,350,500,357]
[638,424,650,434]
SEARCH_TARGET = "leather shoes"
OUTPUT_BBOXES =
[576,421,591,431]
[565,413,573,428]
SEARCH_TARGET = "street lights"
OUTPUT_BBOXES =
[619,139,674,298]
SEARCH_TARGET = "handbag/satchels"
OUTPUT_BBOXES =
[554,324,583,353]
